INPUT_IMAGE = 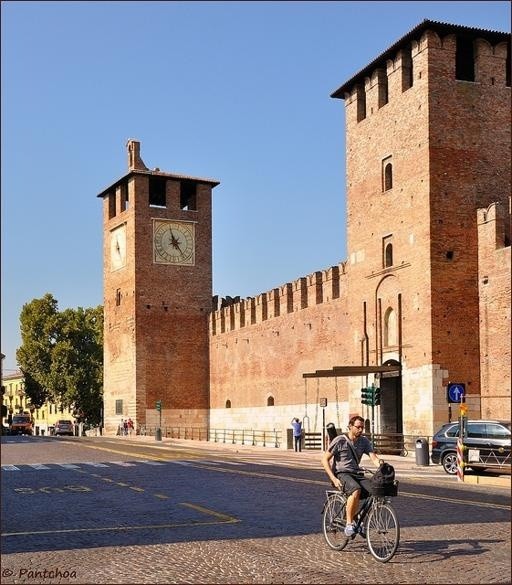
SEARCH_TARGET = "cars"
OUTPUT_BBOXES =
[53,420,75,436]
[50,427,63,436]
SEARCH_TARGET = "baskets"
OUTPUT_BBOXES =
[369,479,399,497]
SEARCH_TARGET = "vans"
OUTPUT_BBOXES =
[10,414,33,436]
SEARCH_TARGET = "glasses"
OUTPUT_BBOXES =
[353,424,365,430]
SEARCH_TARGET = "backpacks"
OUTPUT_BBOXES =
[371,459,395,483]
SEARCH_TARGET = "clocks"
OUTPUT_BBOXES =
[109,221,129,272]
[149,216,198,269]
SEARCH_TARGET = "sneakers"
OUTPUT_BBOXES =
[344,523,357,536]
[357,519,366,534]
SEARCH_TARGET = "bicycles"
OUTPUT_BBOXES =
[319,466,400,563]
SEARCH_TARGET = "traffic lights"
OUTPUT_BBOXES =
[155,400,161,412]
[361,387,372,405]
[372,387,381,406]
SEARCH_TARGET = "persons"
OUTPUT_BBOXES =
[119,419,134,437]
[291,417,302,453]
[321,416,384,538]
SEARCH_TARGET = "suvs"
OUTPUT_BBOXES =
[431,419,511,475]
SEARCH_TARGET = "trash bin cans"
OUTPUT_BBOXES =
[155,428,161,441]
[415,438,429,467]
